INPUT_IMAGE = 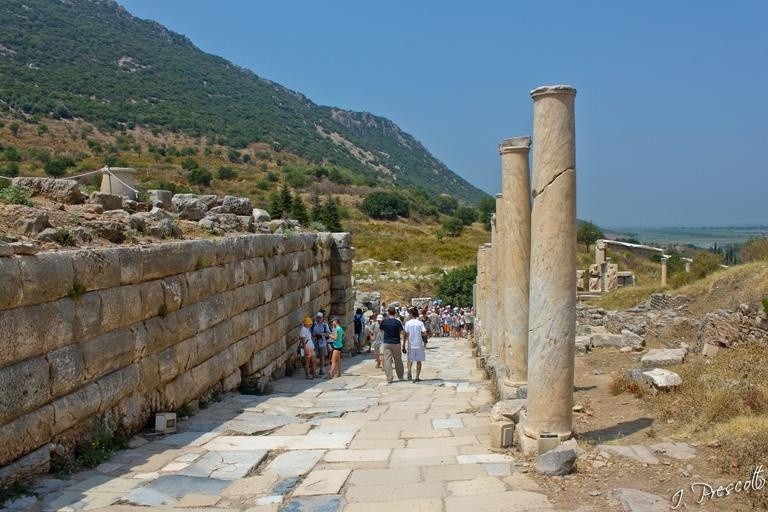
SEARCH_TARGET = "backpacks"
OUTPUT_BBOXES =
[354,315,362,334]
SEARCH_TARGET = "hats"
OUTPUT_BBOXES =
[304,317,313,324]
[330,316,341,321]
[315,312,323,318]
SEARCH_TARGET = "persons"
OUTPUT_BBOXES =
[377,306,404,382]
[402,306,428,382]
[297,309,348,380]
[369,314,384,370]
[353,303,474,350]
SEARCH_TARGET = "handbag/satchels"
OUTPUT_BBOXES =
[297,340,304,357]
